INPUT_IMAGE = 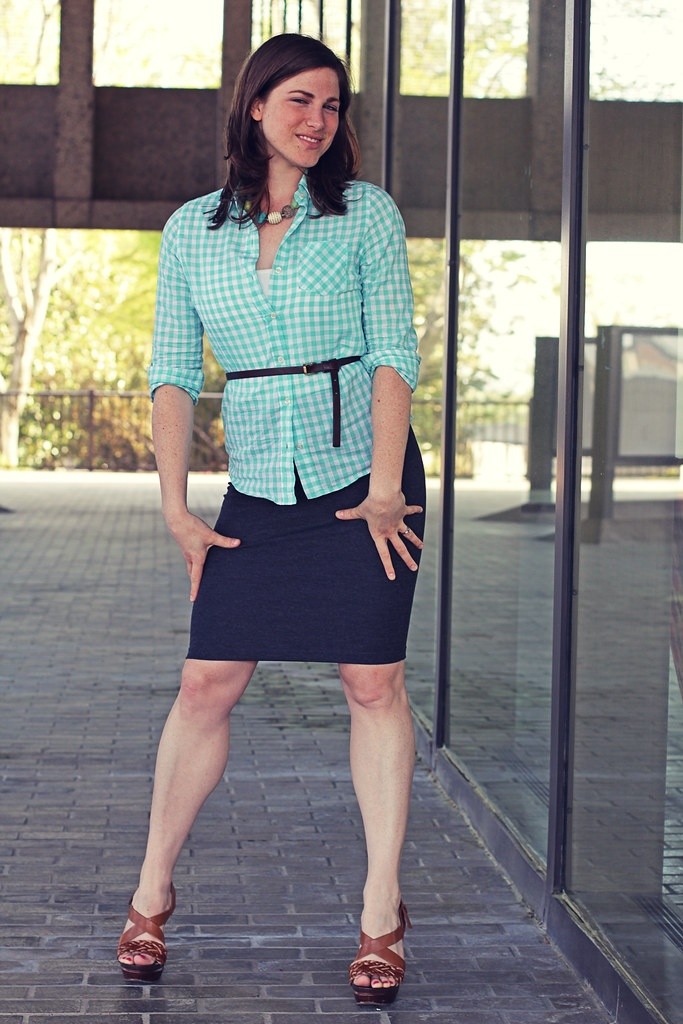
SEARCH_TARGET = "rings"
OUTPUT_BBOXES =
[401,526,410,534]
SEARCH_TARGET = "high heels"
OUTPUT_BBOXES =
[116,882,176,981]
[348,901,412,1004]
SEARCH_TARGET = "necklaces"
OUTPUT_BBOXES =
[244,199,299,224]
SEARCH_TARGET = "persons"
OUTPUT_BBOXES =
[118,34,426,1001]
